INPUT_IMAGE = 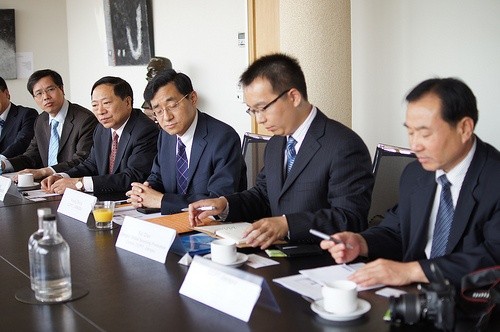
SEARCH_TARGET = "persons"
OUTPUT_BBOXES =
[188,52,375,251]
[140,56,172,109]
[40,76,161,201]
[319,77,500,293]
[0,75,39,159]
[125,69,247,216]
[0,69,99,184]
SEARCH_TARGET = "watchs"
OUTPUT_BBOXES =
[74,177,85,192]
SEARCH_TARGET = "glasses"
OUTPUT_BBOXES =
[246,89,289,115]
[153,93,188,117]
[34,85,57,97]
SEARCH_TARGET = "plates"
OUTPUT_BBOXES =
[205,252,248,267]
[17,182,40,190]
[310,298,370,322]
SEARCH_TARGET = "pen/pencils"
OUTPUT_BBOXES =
[181,205,216,212]
[309,229,353,249]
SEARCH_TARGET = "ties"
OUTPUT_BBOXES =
[176,137,188,193]
[48,120,60,167]
[430,172,454,258]
[108,132,118,173]
[286,136,298,174]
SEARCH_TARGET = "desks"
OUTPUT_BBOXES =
[0,194,500,332]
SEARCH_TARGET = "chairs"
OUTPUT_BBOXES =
[367,142,419,227]
[242,133,274,190]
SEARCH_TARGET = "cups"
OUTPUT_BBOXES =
[320,279,360,313]
[210,239,238,263]
[91,201,115,228]
[17,173,34,187]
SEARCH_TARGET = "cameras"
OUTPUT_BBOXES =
[390,284,458,332]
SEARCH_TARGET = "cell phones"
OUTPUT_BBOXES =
[136,206,161,214]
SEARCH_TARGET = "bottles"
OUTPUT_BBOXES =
[35,214,71,302]
[28,207,63,291]
